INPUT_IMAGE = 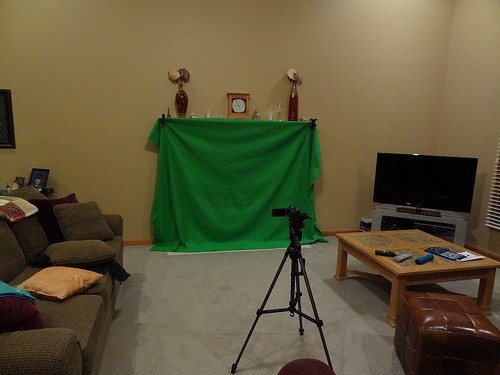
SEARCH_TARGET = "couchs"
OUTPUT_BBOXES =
[0,186,124,375]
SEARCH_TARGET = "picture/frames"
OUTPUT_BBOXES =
[28,168,50,193]
[0,88,16,149]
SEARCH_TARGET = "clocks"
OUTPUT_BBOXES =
[227,93,251,118]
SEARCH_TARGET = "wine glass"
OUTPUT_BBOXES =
[276,103,286,121]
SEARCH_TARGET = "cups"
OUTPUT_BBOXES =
[268,111,273,120]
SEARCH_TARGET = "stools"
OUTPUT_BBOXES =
[394,290,500,375]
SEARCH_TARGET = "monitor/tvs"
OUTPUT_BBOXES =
[372,152,479,219]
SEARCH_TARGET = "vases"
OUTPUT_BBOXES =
[288,81,298,121]
[176,84,188,118]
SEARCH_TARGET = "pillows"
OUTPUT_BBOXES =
[0,193,111,318]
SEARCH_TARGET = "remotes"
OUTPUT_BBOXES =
[394,253,412,262]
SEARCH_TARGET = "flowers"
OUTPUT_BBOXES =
[169,68,190,84]
[287,69,302,86]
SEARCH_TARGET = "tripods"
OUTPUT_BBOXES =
[231,229,333,372]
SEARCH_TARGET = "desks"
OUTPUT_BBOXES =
[334,229,500,328]
[372,206,468,246]
